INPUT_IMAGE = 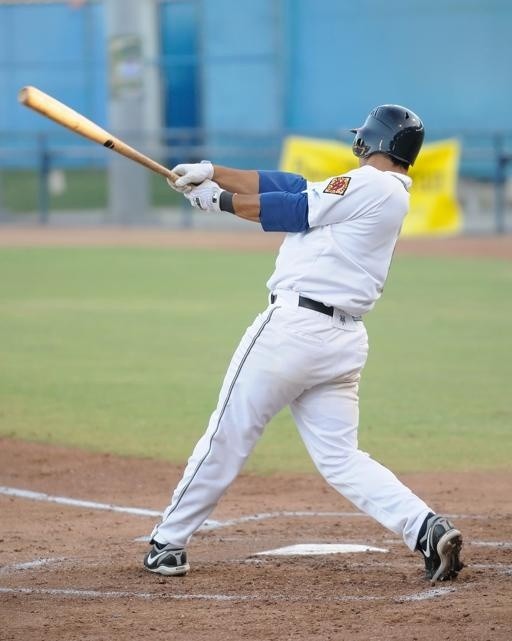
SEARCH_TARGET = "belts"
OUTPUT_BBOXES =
[272,295,362,321]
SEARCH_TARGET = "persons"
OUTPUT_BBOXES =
[141,101,464,583]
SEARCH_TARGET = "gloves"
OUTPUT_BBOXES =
[182,179,236,215]
[167,160,214,193]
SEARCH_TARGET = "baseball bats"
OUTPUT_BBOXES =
[19,87,197,190]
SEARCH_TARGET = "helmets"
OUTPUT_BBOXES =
[348,104,424,166]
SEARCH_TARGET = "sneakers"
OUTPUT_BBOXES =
[418,515,463,583]
[144,541,189,575]
